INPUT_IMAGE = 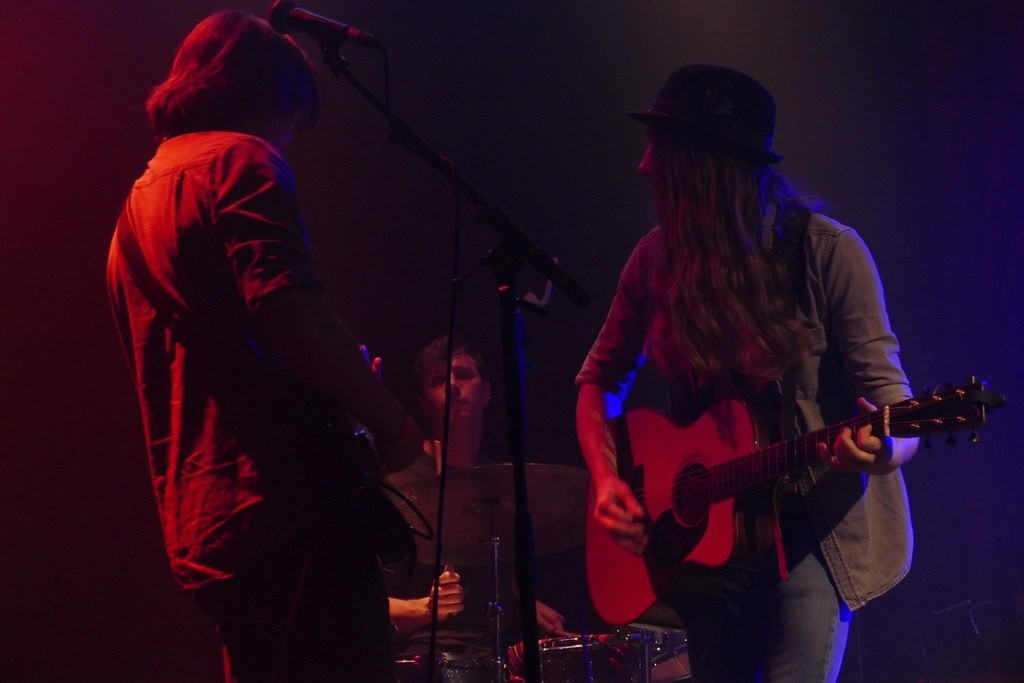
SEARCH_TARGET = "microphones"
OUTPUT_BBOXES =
[266,0,374,45]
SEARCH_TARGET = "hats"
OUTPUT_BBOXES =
[628,64,787,161]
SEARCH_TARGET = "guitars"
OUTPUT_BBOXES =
[582,371,1011,631]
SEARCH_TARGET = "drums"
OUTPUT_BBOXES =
[394,651,508,682]
[537,631,671,683]
[630,641,692,683]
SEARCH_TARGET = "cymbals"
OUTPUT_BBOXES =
[393,461,591,565]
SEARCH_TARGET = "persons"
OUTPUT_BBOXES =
[381,335,562,683]
[105,9,427,683]
[576,65,919,683]
